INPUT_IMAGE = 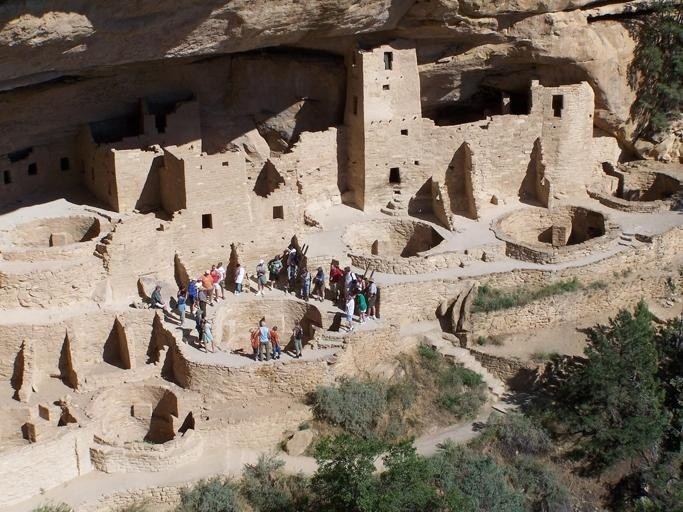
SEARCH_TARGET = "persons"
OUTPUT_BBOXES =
[255,244,378,331]
[232,263,245,292]
[248,327,258,360]
[176,261,226,353]
[268,326,281,360]
[258,318,269,361]
[150,285,168,315]
[292,319,302,358]
